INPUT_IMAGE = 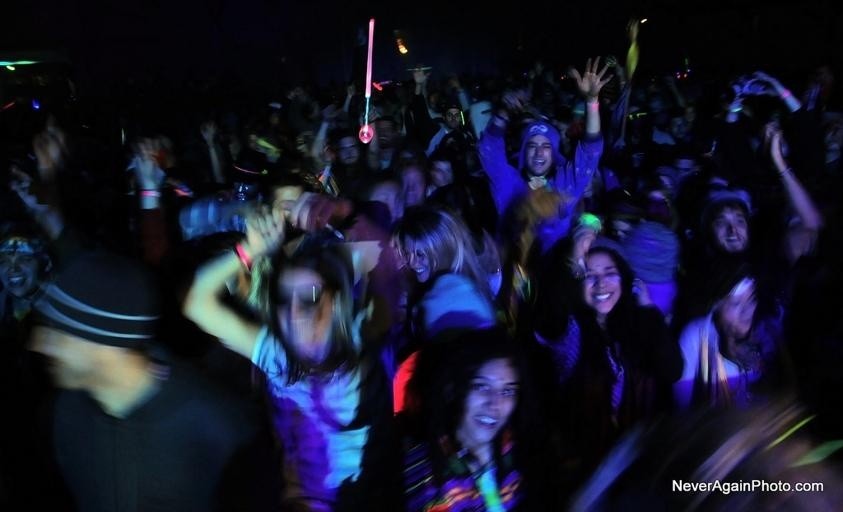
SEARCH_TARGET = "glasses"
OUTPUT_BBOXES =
[268,282,330,310]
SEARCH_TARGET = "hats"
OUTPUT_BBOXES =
[517,120,570,174]
[33,249,160,351]
